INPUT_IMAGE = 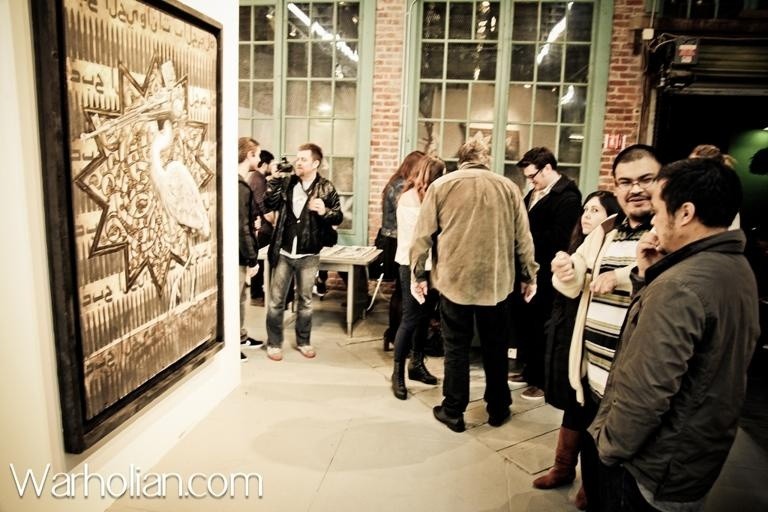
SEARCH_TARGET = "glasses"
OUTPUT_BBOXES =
[524,169,540,178]
[615,174,660,191]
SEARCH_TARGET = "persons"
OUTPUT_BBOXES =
[409,138,540,434]
[508,147,582,405]
[390,157,446,400]
[527,191,623,510]
[372,151,427,348]
[547,142,662,510]
[239,137,264,364]
[244,147,277,306]
[588,155,762,510]
[687,143,741,170]
[258,141,344,362]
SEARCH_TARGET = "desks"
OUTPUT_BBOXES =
[253,244,386,338]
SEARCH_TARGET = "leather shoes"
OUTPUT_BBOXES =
[433,406,465,433]
[488,408,510,425]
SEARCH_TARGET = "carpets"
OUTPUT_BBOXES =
[334,337,565,475]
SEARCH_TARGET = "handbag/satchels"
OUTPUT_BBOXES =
[370,229,398,281]
[239,181,273,250]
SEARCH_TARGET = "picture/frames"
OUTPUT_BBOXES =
[26,0,226,454]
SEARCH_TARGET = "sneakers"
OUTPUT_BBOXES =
[267,346,282,360]
[507,374,528,386]
[297,345,315,357]
[241,352,247,361]
[241,338,264,349]
[521,386,545,400]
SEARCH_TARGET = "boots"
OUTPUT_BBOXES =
[576,482,587,509]
[534,425,579,488]
[408,349,437,384]
[392,359,407,399]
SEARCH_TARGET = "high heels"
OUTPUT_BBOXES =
[383,327,398,350]
